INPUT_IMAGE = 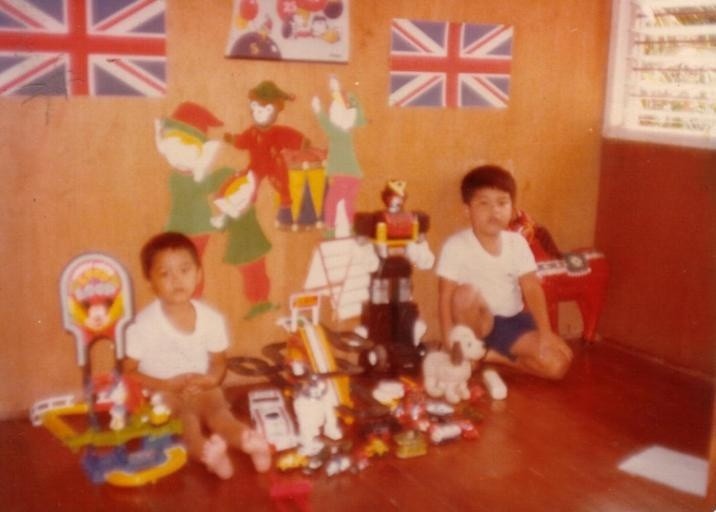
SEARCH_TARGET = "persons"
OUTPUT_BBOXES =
[123,230,272,478]
[356,179,434,378]
[437,165,573,380]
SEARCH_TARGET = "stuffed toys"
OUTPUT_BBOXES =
[395,392,461,443]
[424,325,486,404]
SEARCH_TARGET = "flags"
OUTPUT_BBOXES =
[1,0,167,99]
[387,19,514,112]
